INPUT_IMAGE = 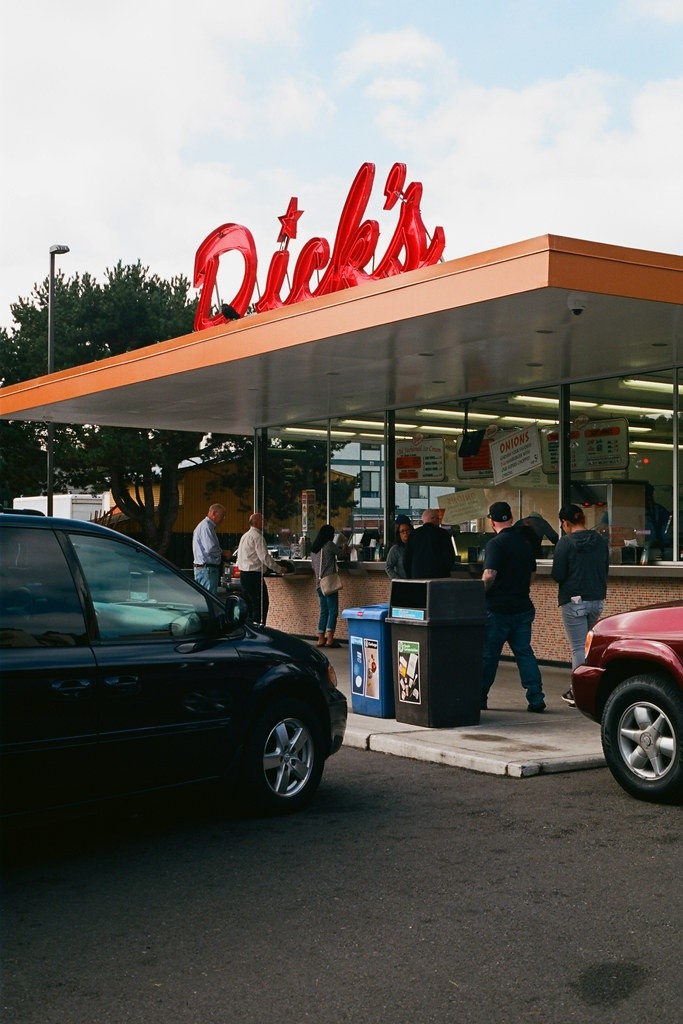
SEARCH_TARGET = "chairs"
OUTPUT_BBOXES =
[31,551,84,615]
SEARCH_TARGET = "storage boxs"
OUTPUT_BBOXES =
[572,503,607,531]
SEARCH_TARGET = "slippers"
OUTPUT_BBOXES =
[325,642,342,647]
[317,640,325,647]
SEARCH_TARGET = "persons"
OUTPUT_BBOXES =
[551,505,609,709]
[310,524,348,648]
[384,514,414,581]
[481,501,548,713]
[512,512,559,559]
[406,509,456,579]
[192,504,231,597]
[237,513,288,626]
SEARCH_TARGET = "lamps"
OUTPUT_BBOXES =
[629,436,683,451]
[617,373,683,395]
[595,399,675,420]
[359,431,413,441]
[278,423,358,439]
[627,420,655,433]
[499,412,555,426]
[418,422,478,436]
[413,404,502,423]
[336,415,418,431]
[507,390,603,411]
[454,400,486,458]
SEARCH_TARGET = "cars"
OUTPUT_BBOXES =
[0,510,349,819]
[560,601,683,804]
[220,544,292,594]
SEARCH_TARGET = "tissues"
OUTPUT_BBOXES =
[620,538,645,566]
[364,539,377,561]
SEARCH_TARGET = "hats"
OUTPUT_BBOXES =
[526,512,542,518]
[487,502,512,522]
[559,504,583,521]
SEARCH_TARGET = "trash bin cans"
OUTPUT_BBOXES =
[385,578,488,729]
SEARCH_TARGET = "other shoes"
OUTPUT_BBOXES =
[480,697,488,709]
[527,701,546,710]
[561,689,575,704]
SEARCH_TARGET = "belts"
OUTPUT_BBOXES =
[194,564,217,567]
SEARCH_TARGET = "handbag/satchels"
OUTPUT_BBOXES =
[320,574,343,596]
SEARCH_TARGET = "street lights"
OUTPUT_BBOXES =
[47,246,69,518]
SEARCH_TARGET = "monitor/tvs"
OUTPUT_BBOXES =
[458,430,487,458]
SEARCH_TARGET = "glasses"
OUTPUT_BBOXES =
[399,529,411,534]
